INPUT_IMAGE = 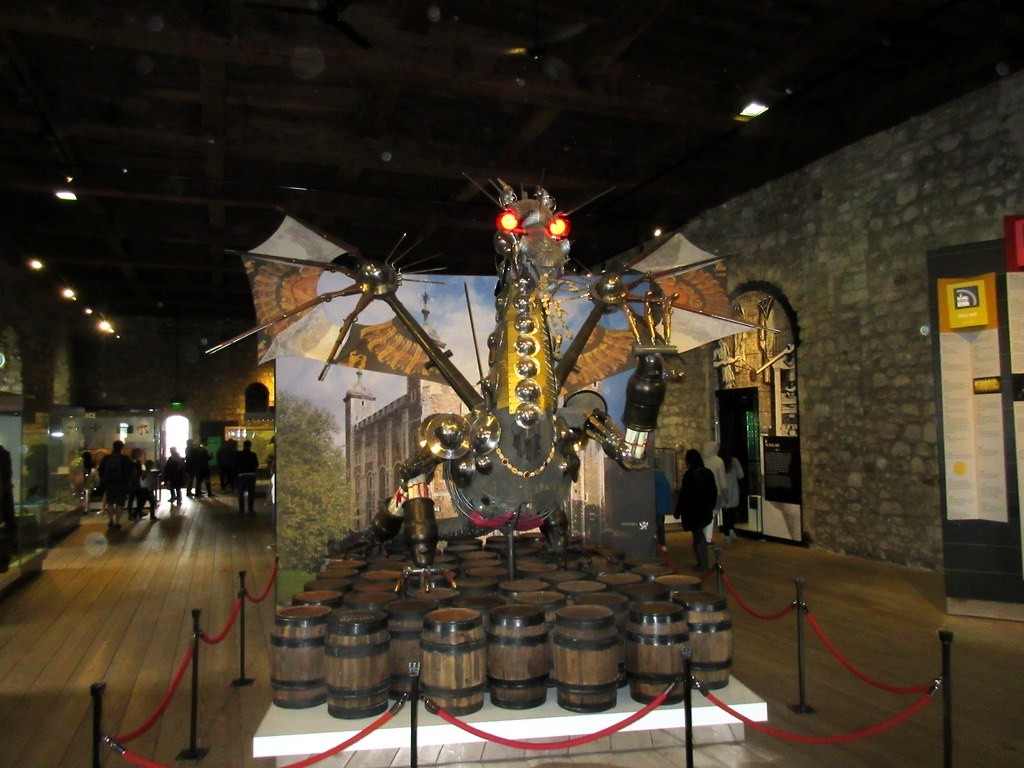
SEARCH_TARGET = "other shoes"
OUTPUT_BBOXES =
[693,562,709,570]
[151,516,157,521]
[187,492,194,496]
[208,493,215,497]
[108,520,121,529]
[661,545,668,552]
[201,491,205,494]
[726,530,737,543]
[128,515,136,521]
[96,510,104,515]
[141,512,148,516]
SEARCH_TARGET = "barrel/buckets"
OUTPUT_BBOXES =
[269,531,732,721]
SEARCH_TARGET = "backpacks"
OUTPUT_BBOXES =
[104,456,122,481]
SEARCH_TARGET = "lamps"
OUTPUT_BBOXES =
[507,47,548,64]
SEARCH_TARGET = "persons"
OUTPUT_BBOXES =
[711,338,742,390]
[716,436,744,542]
[76,434,276,529]
[672,448,719,572]
[700,441,730,547]
[652,458,673,553]
